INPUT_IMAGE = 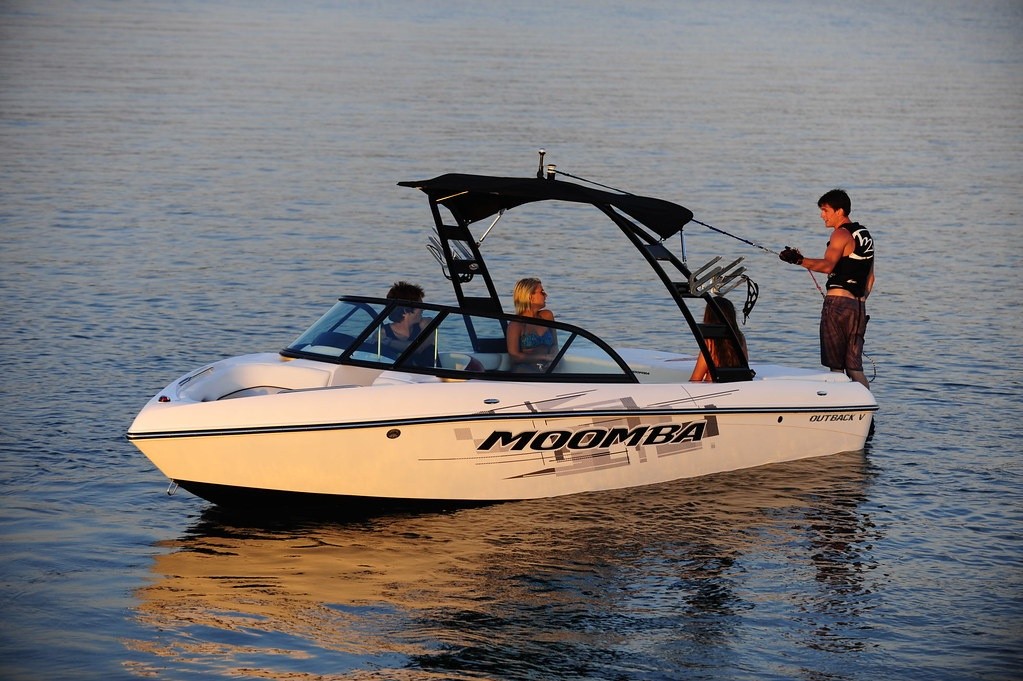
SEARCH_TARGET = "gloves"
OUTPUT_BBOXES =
[779,246,804,266]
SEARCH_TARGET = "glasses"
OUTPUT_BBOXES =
[534,289,545,296]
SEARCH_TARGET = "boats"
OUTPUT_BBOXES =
[124,146,879,501]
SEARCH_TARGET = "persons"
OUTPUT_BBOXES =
[779,189,875,391]
[371,282,443,368]
[690,296,749,383]
[507,277,561,373]
